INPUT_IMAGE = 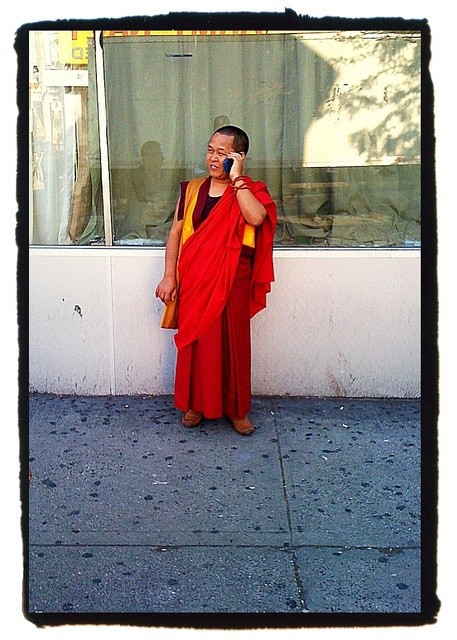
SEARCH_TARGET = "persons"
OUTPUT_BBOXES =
[214,114,229,130]
[155,125,277,434]
[118,140,172,236]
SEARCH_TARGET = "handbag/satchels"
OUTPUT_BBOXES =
[159,176,208,330]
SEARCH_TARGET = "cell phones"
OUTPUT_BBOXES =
[222,153,241,173]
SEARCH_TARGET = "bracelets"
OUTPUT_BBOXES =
[230,176,249,193]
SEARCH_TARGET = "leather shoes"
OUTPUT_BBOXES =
[234,416,253,435]
[182,410,201,427]
